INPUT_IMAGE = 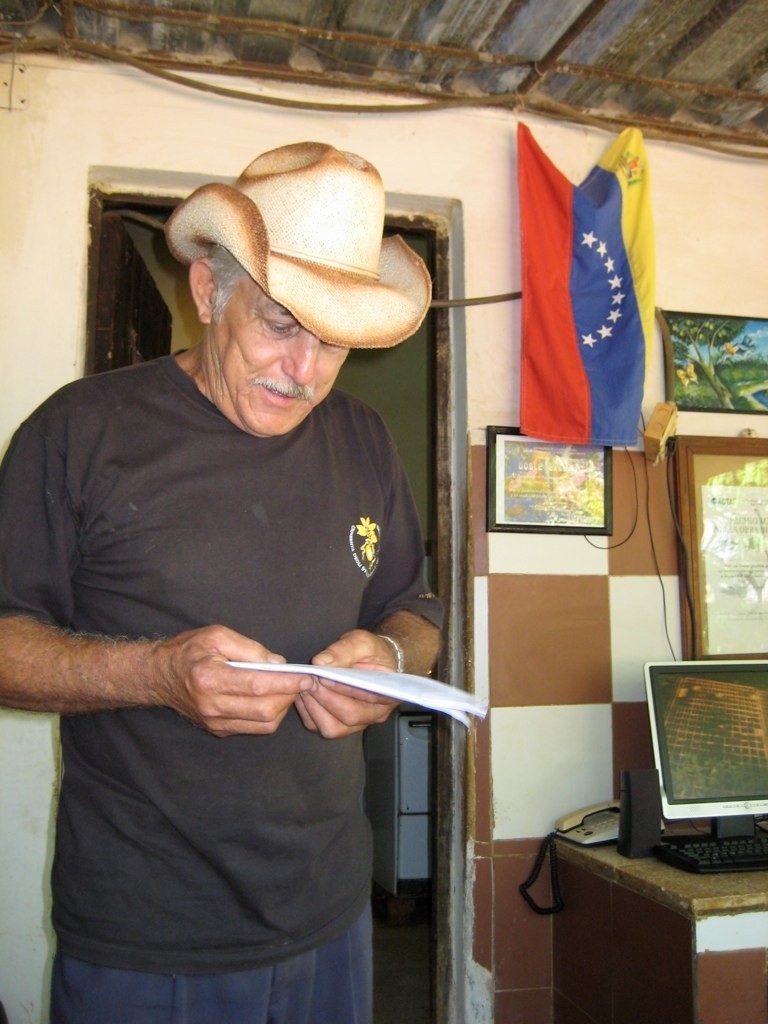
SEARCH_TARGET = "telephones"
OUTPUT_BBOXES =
[555,800,623,846]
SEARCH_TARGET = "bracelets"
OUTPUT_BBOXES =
[378,634,405,674]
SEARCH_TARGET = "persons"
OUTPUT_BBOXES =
[0,140,448,1023]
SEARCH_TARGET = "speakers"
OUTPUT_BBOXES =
[618,769,660,857]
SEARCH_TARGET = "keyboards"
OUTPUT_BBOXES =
[656,838,768,874]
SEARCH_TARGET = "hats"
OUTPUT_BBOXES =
[166,142,430,348]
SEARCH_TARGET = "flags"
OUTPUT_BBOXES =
[518,121,657,448]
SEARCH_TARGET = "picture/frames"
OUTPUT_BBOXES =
[657,308,768,413]
[484,425,615,541]
[674,435,768,662]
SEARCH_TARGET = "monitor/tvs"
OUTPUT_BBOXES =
[644,660,768,842]
